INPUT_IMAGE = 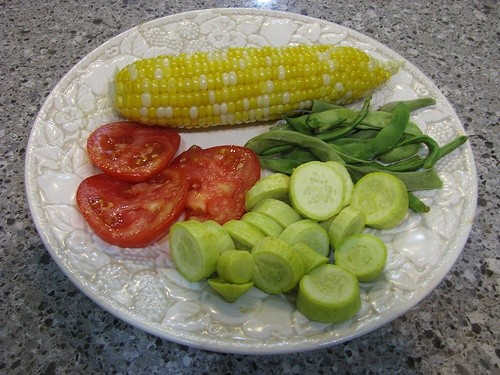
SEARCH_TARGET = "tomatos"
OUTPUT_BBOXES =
[76,121,260,248]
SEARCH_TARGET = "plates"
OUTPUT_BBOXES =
[25,8,479,354]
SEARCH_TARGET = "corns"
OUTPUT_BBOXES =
[110,43,405,128]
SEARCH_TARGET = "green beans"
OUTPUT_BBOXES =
[244,92,468,212]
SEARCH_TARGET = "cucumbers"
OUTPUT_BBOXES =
[168,160,410,323]
